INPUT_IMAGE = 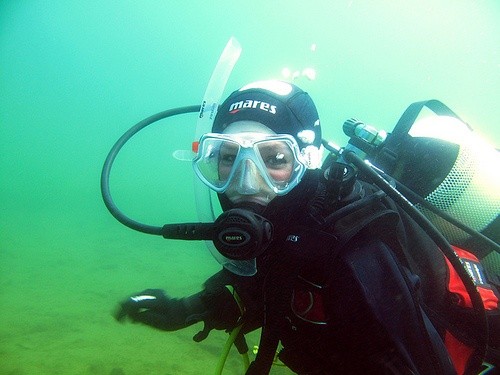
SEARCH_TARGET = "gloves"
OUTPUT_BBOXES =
[116,289,225,331]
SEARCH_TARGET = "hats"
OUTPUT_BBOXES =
[212,79,322,152]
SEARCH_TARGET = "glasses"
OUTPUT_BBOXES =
[191,133,307,196]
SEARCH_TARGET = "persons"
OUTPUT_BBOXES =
[113,81,500,375]
